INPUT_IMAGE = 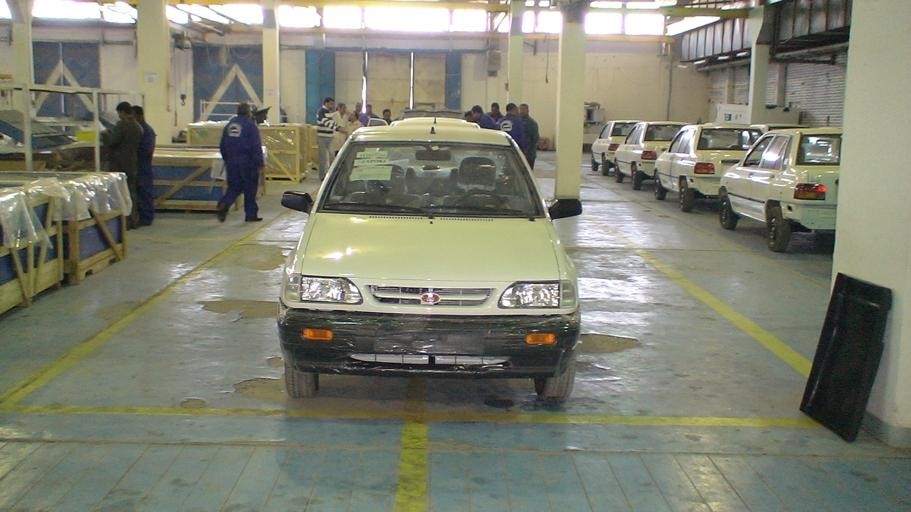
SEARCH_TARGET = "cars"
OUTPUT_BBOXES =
[750,123,811,136]
[276,126,583,406]
[591,120,646,176]
[718,126,844,253]
[654,123,771,213]
[367,109,481,130]
[614,121,689,190]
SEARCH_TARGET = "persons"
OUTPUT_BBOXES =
[132,104,158,227]
[461,100,541,174]
[216,102,266,224]
[97,99,146,231]
[315,96,394,181]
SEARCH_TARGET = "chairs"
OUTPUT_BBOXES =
[645,130,653,141]
[349,156,498,207]
[610,127,621,135]
[696,136,708,149]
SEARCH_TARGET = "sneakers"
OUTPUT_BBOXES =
[216,200,229,221]
[245,212,263,221]
[125,218,153,230]
